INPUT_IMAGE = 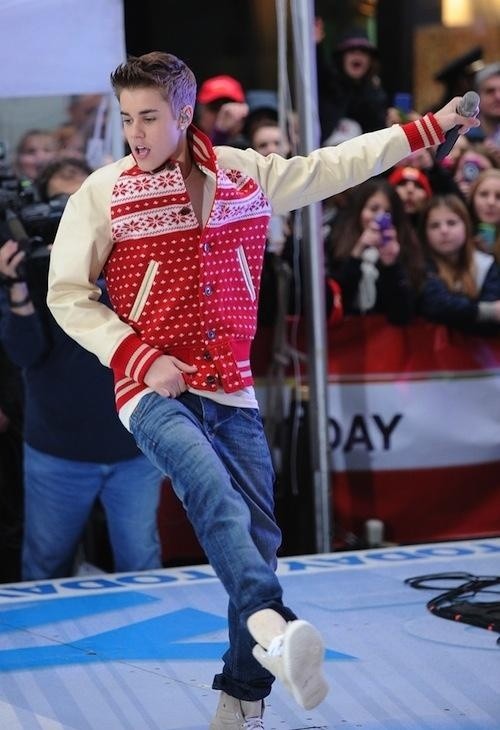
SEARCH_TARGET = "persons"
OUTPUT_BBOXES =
[2,75,301,328]
[0,154,166,581]
[309,0,500,328]
[45,48,482,730]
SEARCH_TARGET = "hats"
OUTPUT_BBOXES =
[198,77,244,104]
[340,36,372,56]
[389,168,434,199]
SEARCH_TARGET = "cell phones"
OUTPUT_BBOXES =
[374,211,392,243]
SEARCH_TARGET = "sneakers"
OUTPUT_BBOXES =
[253,620,328,711]
[214,691,266,730]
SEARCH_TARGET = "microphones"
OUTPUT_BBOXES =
[435,90,481,161]
